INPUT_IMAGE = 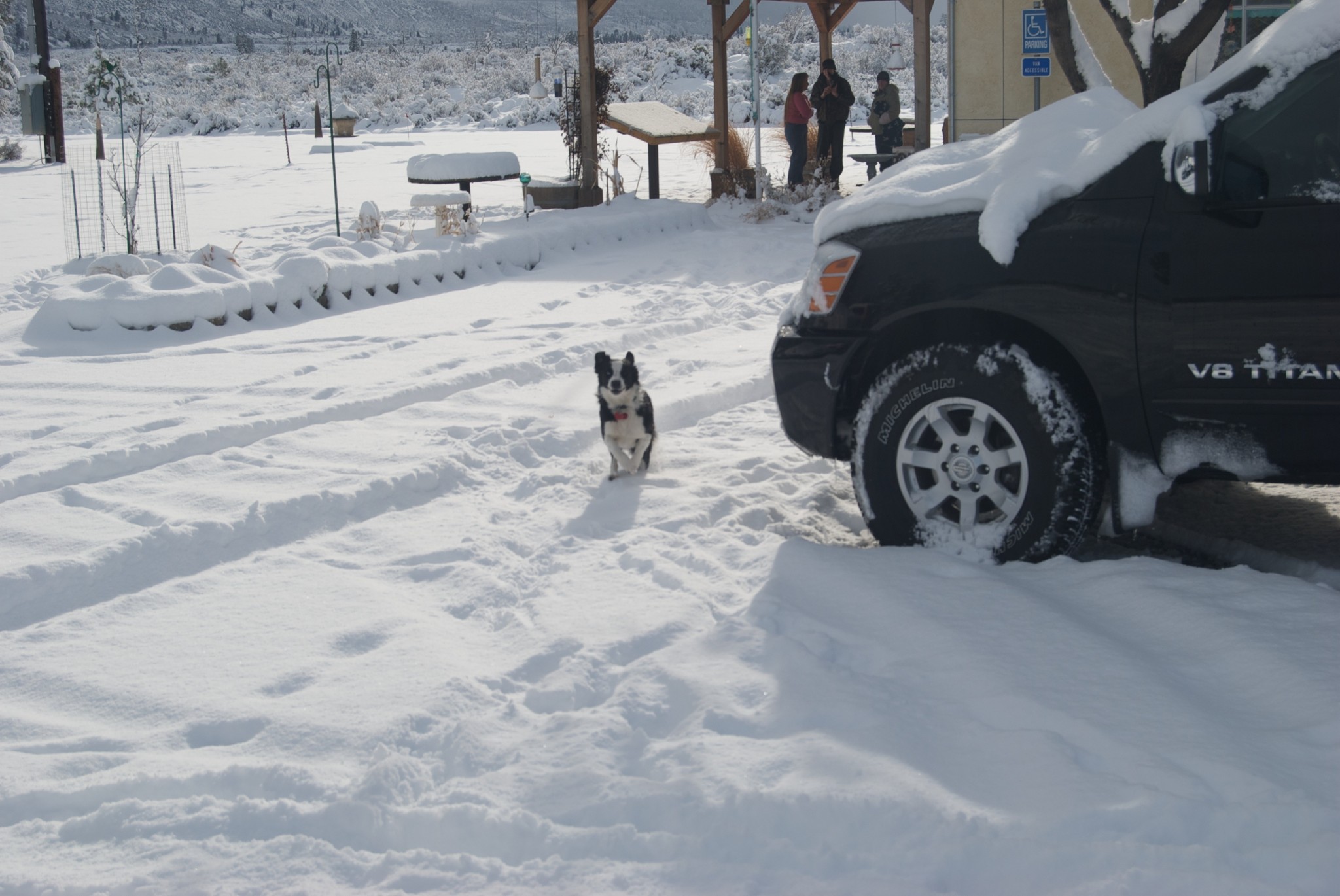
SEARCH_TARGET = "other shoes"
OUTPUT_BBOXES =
[831,177,840,190]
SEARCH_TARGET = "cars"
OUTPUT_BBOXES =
[770,0,1340,579]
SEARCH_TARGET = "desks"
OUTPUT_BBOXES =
[850,124,915,133]
[407,173,522,224]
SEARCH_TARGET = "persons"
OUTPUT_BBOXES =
[784,72,814,187]
[867,71,903,172]
[809,59,855,189]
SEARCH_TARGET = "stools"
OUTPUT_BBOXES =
[411,189,471,234]
[846,153,898,183]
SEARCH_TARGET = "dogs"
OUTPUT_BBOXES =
[594,350,656,480]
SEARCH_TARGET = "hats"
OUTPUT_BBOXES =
[877,70,890,82]
[822,58,837,71]
